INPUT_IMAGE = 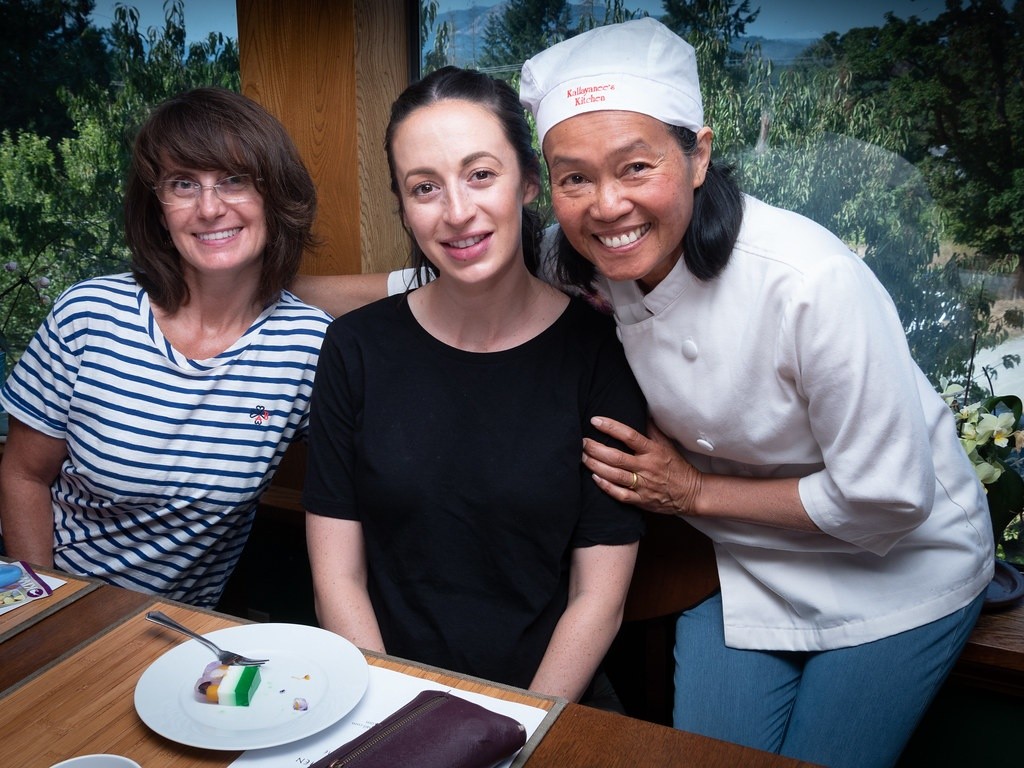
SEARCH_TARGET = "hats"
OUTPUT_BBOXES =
[519,17,703,133]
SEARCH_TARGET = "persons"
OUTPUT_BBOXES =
[0,85,335,611]
[292,65,649,703]
[283,16,998,768]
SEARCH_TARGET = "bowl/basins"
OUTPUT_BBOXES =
[48,753,141,768]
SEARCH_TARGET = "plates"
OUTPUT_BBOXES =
[134,623,369,751]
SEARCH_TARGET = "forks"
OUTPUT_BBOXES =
[145,609,269,667]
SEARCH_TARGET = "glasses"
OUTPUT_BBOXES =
[153,173,266,205]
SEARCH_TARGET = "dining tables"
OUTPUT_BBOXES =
[0,556,806,768]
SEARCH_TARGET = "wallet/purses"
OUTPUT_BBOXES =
[306,690,527,768]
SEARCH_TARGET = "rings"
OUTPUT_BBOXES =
[629,473,638,489]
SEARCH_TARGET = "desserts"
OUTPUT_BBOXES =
[195,660,261,706]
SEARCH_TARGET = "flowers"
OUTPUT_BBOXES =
[939,384,1024,557]
